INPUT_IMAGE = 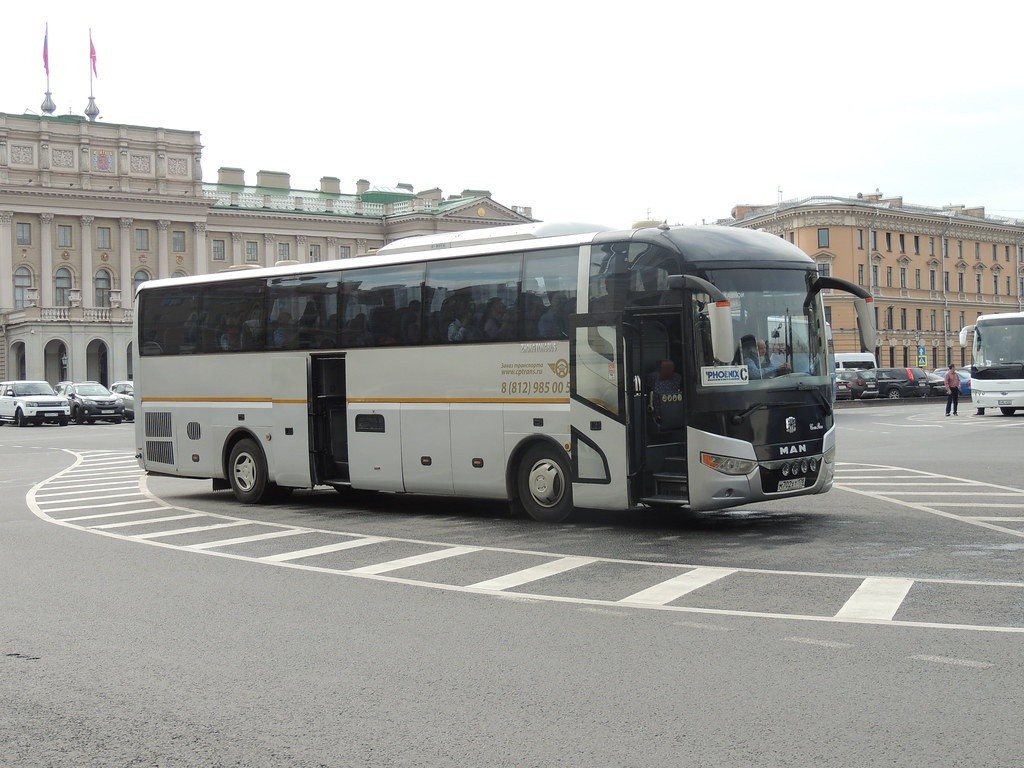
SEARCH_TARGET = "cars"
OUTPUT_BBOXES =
[926,372,948,397]
[935,370,971,397]
[832,378,852,400]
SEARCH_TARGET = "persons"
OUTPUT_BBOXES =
[944,363,961,416]
[743,338,792,380]
[214,293,569,351]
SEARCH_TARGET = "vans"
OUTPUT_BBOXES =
[832,352,877,372]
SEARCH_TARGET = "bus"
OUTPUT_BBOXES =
[134,210,879,528]
[958,312,1024,417]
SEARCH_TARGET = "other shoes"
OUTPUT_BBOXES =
[946,413,958,416]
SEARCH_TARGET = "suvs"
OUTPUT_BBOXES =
[111,380,134,421]
[836,367,879,399]
[0,379,71,427]
[867,368,930,400]
[54,380,126,424]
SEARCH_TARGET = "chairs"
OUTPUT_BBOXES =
[145,293,575,353]
[646,360,682,430]
